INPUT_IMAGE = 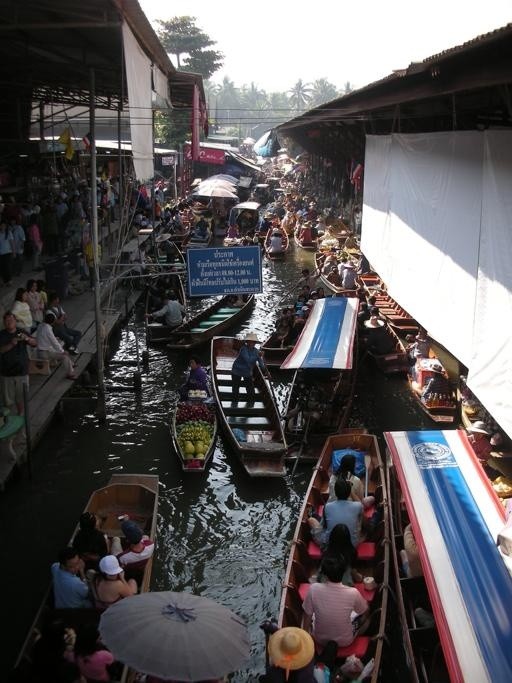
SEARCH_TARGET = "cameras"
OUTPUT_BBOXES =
[117,516,126,522]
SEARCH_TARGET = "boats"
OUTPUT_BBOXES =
[269,429,393,683]
[381,425,511,683]
[6,469,165,683]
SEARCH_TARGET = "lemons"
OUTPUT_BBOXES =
[176,419,214,437]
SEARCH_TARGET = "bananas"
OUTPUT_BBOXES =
[178,427,211,448]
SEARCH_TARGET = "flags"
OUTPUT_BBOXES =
[82,132,94,153]
[56,125,75,159]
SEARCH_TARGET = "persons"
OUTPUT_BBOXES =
[2,148,495,682]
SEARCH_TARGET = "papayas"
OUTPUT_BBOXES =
[183,441,208,459]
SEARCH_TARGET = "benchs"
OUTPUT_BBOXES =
[26,330,66,375]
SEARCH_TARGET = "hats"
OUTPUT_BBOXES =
[327,256,334,262]
[286,193,325,229]
[99,556,123,575]
[121,519,143,543]
[464,421,492,435]
[294,285,318,316]
[268,627,314,670]
[343,260,358,268]
[427,364,445,375]
[363,316,384,328]
[155,234,171,242]
[241,334,263,344]
[272,227,282,234]
